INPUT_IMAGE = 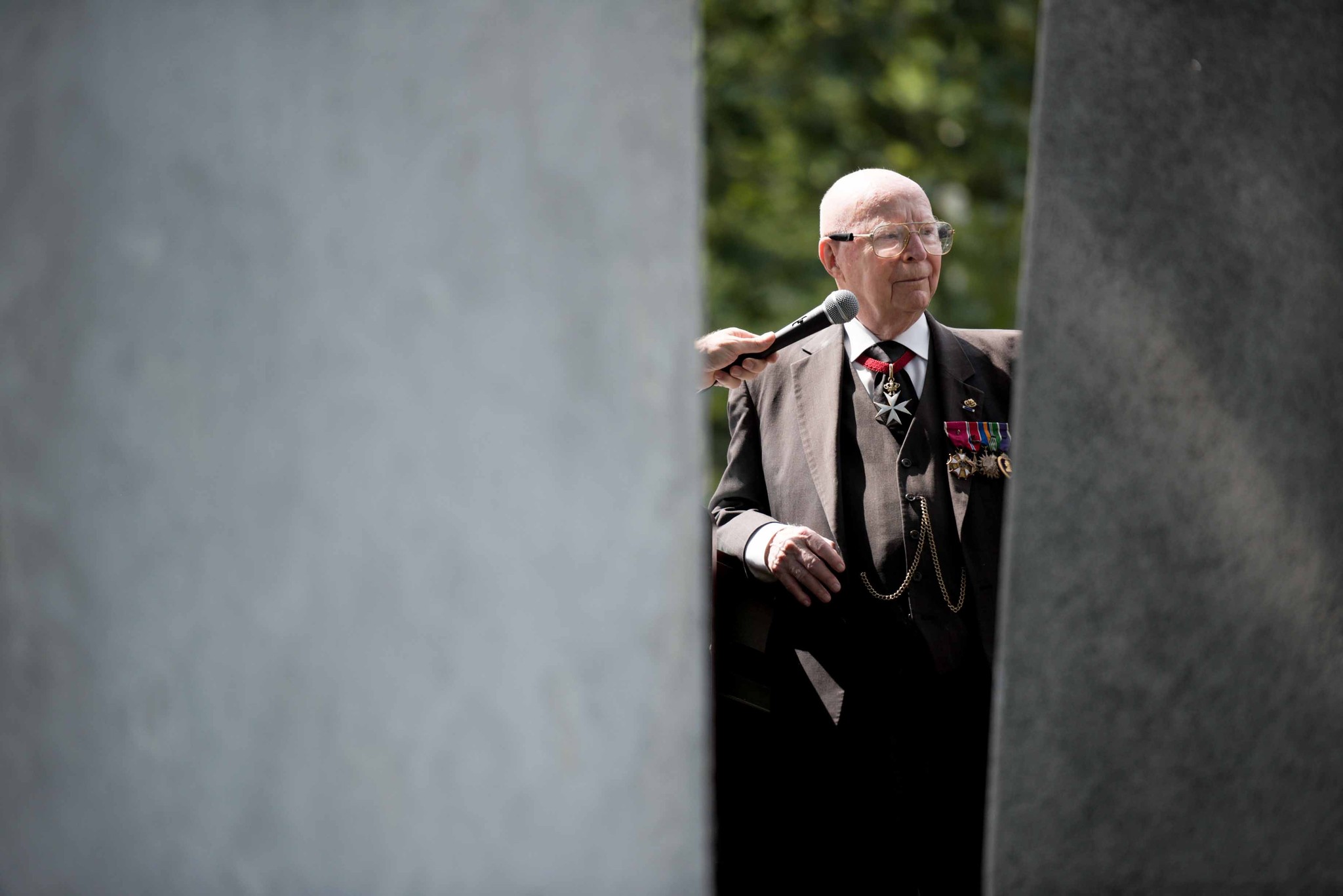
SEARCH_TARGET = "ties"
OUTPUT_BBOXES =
[865,340,919,445]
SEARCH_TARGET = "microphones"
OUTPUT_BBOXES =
[718,290,859,376]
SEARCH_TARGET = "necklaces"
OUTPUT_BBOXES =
[856,350,916,425]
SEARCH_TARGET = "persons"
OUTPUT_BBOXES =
[709,168,1016,896]
[699,327,778,391]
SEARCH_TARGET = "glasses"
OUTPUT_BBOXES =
[824,220,955,258]
